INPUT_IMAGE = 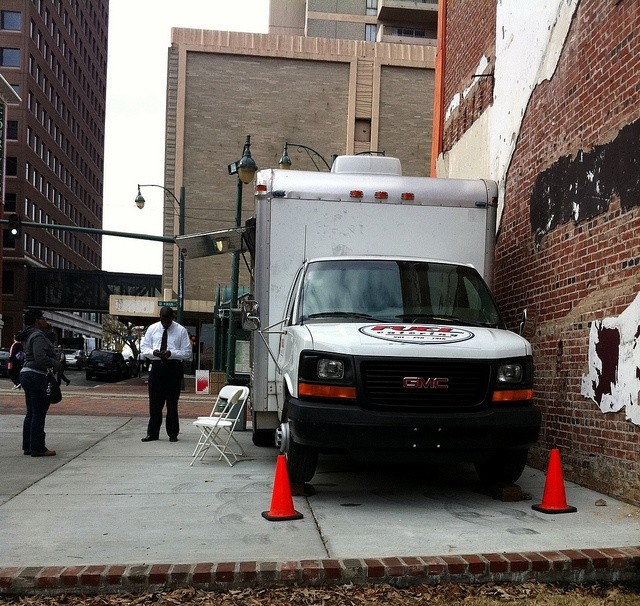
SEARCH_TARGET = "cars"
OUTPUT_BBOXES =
[61,348,86,370]
[86,347,128,382]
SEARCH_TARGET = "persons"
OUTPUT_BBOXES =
[56,344,70,387]
[7,334,23,391]
[140,306,194,442]
[19,308,61,457]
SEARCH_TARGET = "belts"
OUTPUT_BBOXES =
[151,359,182,364]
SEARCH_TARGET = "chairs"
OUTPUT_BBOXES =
[192,386,249,460]
[190,389,243,467]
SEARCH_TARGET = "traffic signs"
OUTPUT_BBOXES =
[157,300,178,308]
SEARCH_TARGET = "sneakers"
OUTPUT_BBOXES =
[32,449,56,456]
[12,383,21,389]
[15,388,21,390]
[24,450,31,455]
[66,380,70,386]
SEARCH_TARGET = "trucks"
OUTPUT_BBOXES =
[249,158,544,482]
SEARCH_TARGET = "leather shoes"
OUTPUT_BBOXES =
[141,436,159,441]
[169,437,177,441]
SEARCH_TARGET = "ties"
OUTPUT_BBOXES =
[160,328,167,351]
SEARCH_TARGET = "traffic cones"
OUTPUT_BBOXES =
[262,454,304,521]
[532,446,578,514]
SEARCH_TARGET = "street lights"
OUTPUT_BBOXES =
[226,133,257,384]
[136,182,186,324]
[277,141,331,171]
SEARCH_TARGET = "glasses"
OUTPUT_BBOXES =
[38,317,46,320]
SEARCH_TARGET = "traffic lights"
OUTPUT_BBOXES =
[7,211,20,238]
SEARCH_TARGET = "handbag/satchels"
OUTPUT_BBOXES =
[41,359,61,404]
